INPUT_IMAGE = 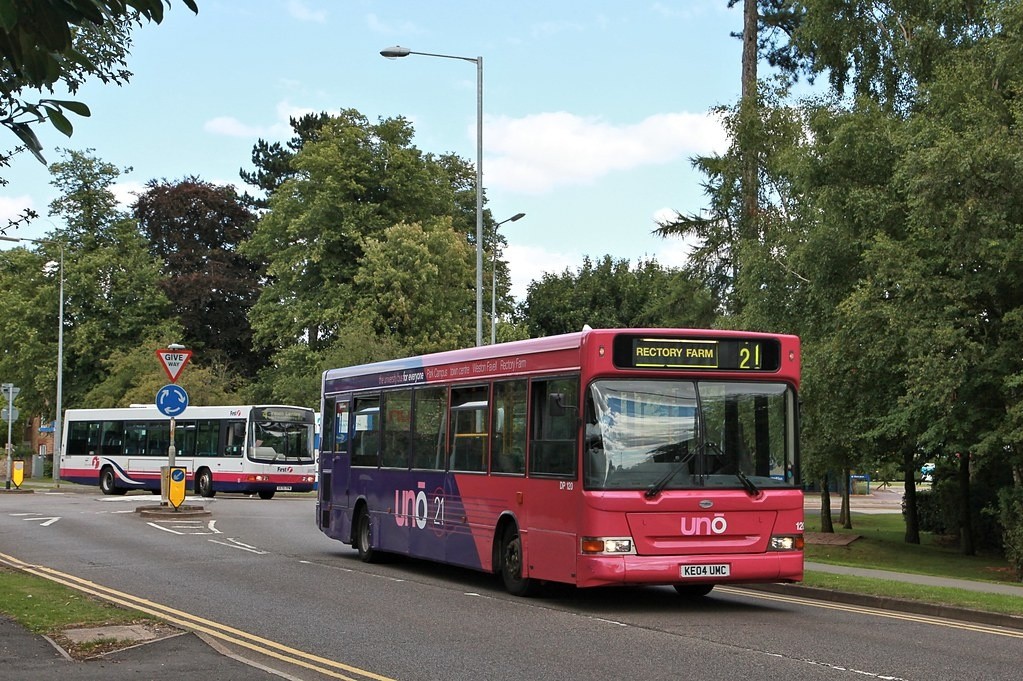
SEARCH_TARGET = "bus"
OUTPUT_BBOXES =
[58,403,316,501]
[312,325,806,600]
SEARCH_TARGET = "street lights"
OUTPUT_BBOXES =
[0,236,64,484]
[378,42,485,346]
[489,210,526,343]
[2,382,16,490]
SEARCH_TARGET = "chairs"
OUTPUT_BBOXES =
[77,435,218,457]
[338,430,568,475]
